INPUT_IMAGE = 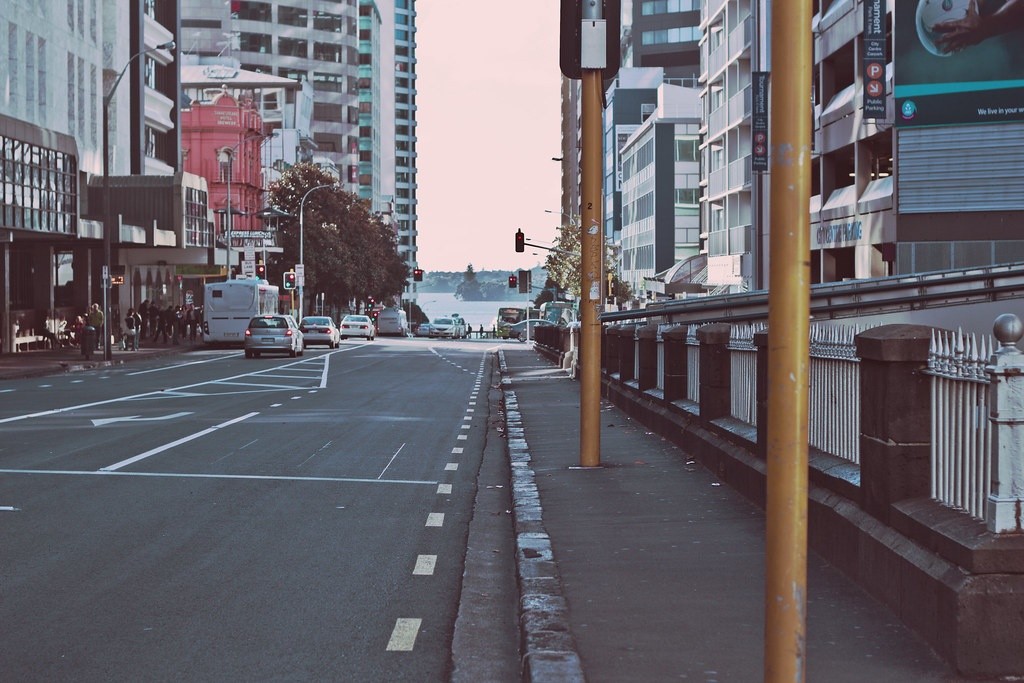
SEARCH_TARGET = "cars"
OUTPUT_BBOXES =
[415,324,433,337]
[298,316,341,349]
[244,314,304,359]
[509,319,557,343]
[340,315,375,341]
[430,317,467,338]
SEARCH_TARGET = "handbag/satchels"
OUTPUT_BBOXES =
[126,328,137,336]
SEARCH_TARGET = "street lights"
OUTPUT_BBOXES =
[102,42,177,361]
[545,210,576,224]
[227,133,279,280]
[421,301,436,323]
[299,184,338,325]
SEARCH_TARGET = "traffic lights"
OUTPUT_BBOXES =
[509,276,517,288]
[256,264,266,280]
[284,272,296,289]
[414,269,423,281]
[516,232,524,252]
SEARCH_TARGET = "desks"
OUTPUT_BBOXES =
[16,336,43,351]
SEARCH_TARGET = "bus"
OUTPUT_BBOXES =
[539,302,573,325]
[377,308,410,338]
[203,279,280,349]
[497,307,541,340]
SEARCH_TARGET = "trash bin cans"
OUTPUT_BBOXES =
[82,326,96,360]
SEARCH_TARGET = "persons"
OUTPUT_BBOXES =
[120,308,141,351]
[466,323,472,339]
[87,304,104,350]
[73,316,83,348]
[932,0,1024,55]
[39,315,64,350]
[493,324,495,339]
[139,299,203,345]
[480,324,483,339]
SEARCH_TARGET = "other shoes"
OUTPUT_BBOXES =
[97,345,101,350]
[138,332,196,346]
[131,347,136,351]
[124,347,128,351]
